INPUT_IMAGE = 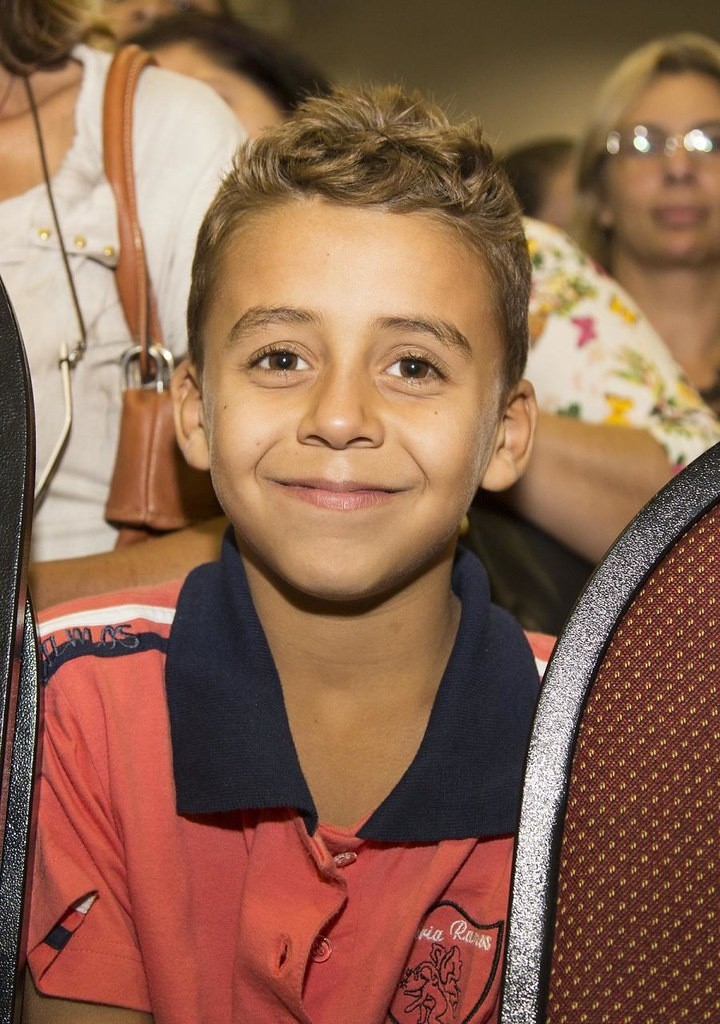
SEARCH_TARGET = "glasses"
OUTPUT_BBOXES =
[607,122,720,159]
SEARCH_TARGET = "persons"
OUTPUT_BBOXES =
[3,2,719,611]
[8,81,556,1024]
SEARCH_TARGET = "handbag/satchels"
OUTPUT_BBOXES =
[103,386,223,532]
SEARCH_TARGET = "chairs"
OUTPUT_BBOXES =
[494,441,720,1024]
